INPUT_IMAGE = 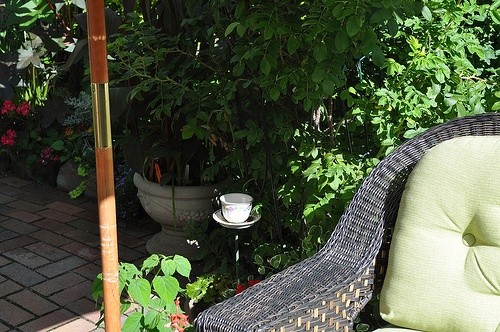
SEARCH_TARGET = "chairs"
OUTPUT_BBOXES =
[191,114,499,331]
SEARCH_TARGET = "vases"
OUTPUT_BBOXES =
[218,193,253,224]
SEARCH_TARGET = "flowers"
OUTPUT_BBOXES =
[0,32,59,163]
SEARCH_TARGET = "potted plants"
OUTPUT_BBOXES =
[134,0,251,265]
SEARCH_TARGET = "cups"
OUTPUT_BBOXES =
[220,193,253,222]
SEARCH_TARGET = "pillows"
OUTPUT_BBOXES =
[378,135,500,331]
